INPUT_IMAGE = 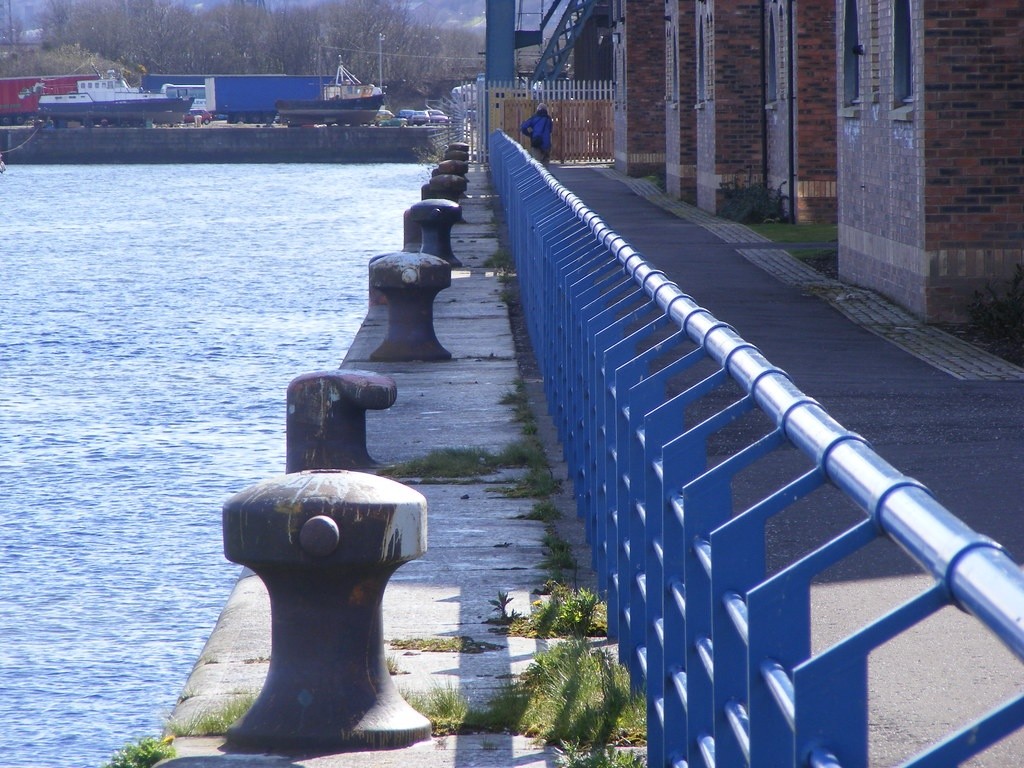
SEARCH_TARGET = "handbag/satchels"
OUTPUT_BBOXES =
[531,136,542,147]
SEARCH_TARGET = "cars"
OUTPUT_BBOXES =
[373,109,449,127]
[185,108,212,125]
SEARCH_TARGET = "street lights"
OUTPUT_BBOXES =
[379,32,385,91]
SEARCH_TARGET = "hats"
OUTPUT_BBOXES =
[537,103,547,113]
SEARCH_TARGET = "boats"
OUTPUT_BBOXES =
[161,83,207,114]
[273,55,386,126]
[38,53,195,126]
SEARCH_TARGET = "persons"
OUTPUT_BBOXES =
[522,103,552,167]
